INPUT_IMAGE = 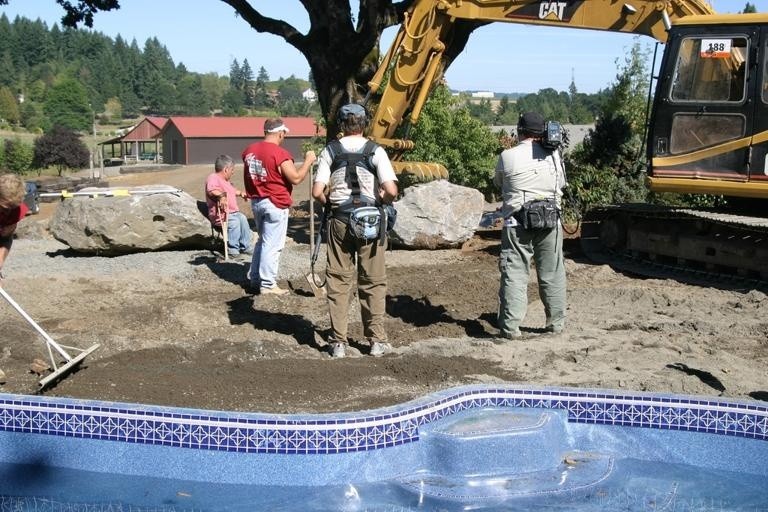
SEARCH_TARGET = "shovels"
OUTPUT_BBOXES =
[304,163,327,298]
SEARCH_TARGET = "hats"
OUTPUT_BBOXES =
[339,103,366,120]
[518,111,545,134]
[267,124,289,135]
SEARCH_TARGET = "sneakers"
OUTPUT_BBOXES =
[260,284,291,297]
[501,328,521,339]
[369,342,393,358]
[329,342,346,358]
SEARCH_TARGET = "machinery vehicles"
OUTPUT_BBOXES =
[339,0,767,290]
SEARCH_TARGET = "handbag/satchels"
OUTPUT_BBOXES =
[348,206,388,240]
[518,198,559,230]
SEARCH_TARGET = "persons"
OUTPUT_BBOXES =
[0,175,28,288]
[312,103,400,358]
[243,118,316,294]
[492,114,567,338]
[204,153,251,259]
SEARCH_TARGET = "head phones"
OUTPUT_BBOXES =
[517,110,548,136]
[336,104,371,129]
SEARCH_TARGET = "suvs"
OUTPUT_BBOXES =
[22,181,41,214]
